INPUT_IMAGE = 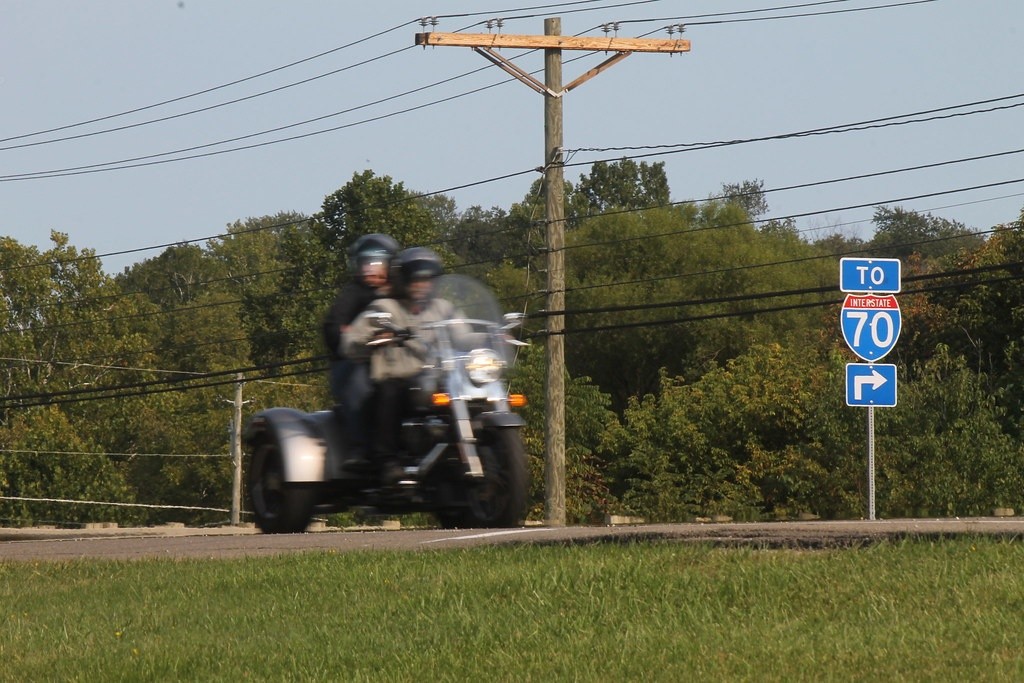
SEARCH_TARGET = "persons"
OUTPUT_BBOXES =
[322,231,515,489]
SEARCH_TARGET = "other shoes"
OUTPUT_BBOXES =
[398,465,420,485]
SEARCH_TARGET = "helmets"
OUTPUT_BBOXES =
[390,247,441,314]
[345,233,399,307]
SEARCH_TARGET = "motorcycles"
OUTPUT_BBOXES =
[243,269,531,533]
